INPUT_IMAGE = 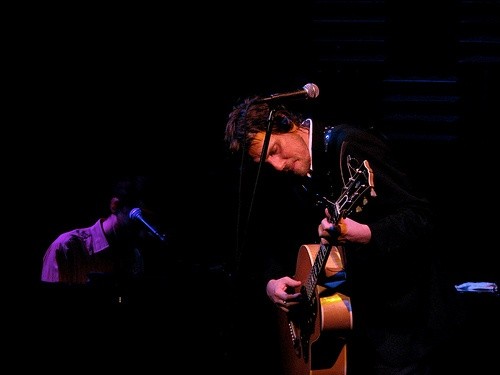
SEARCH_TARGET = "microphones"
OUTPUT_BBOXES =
[253,83,320,104]
[129,208,165,241]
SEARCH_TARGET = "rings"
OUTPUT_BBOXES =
[283,301,287,305]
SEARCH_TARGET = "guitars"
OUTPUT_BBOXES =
[275,154,377,375]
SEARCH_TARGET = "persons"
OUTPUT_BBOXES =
[40,182,145,283]
[224,97,425,375]
